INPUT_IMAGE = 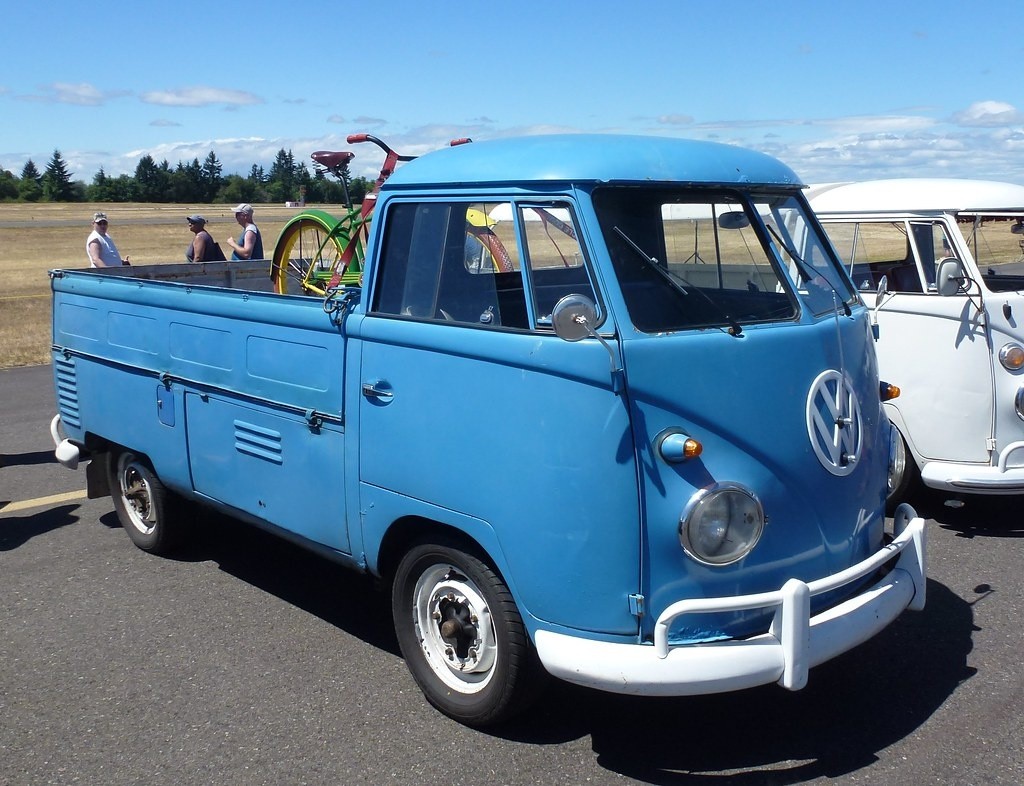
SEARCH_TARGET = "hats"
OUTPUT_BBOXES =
[231,203,253,214]
[187,215,206,225]
[93,212,108,222]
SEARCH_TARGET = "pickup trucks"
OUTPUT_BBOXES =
[47,130,936,730]
[541,176,1024,500]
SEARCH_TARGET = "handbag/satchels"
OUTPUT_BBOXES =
[214,242,227,261]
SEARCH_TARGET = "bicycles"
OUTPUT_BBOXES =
[267,133,513,299]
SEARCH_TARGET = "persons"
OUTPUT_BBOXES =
[185,215,215,263]
[226,203,265,261]
[942,232,952,259]
[85,212,131,269]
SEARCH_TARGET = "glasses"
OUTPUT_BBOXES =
[97,221,107,225]
